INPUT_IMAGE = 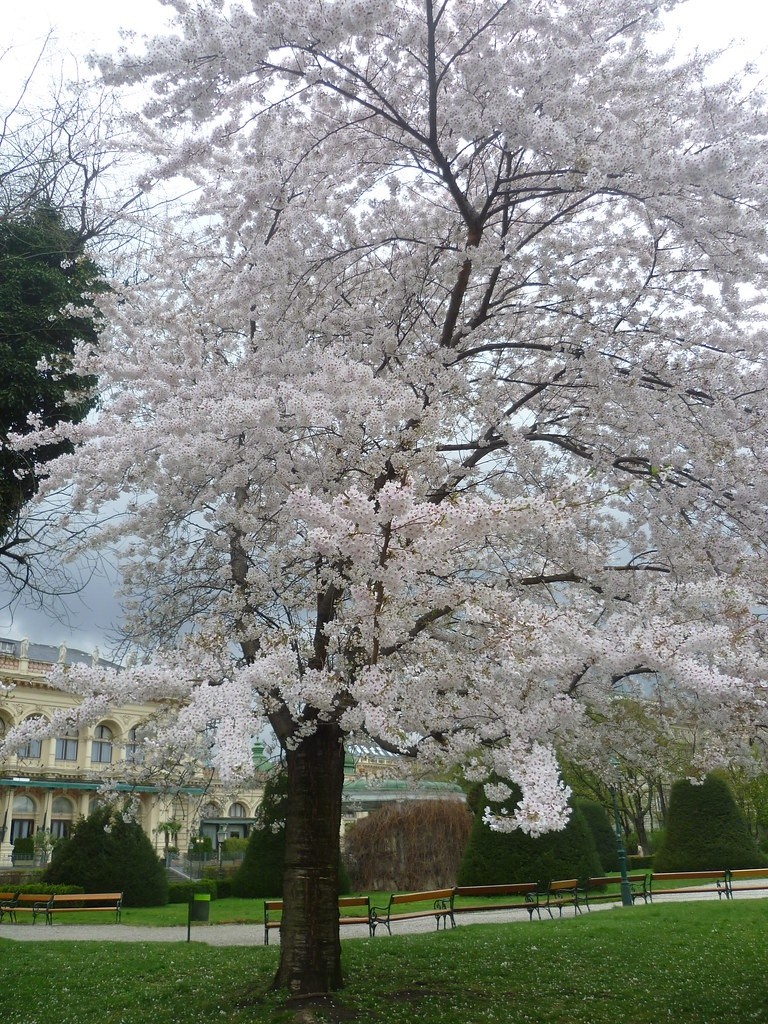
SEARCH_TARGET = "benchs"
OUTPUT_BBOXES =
[1,868,768,945]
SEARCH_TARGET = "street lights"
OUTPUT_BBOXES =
[216,825,228,868]
